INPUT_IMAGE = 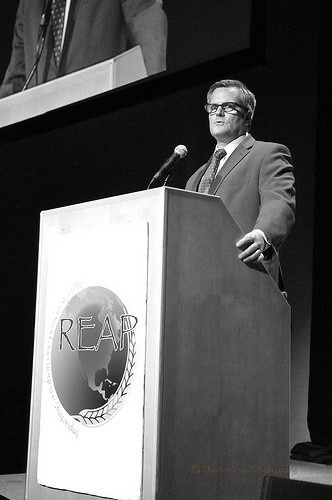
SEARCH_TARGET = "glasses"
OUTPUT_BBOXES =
[205,102,247,114]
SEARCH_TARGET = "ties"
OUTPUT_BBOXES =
[197,148,227,195]
[51,0,66,68]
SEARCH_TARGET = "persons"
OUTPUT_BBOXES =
[184,79,297,296]
[1,0,168,98]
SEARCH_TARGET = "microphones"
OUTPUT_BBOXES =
[39,0,54,34]
[150,145,187,183]
